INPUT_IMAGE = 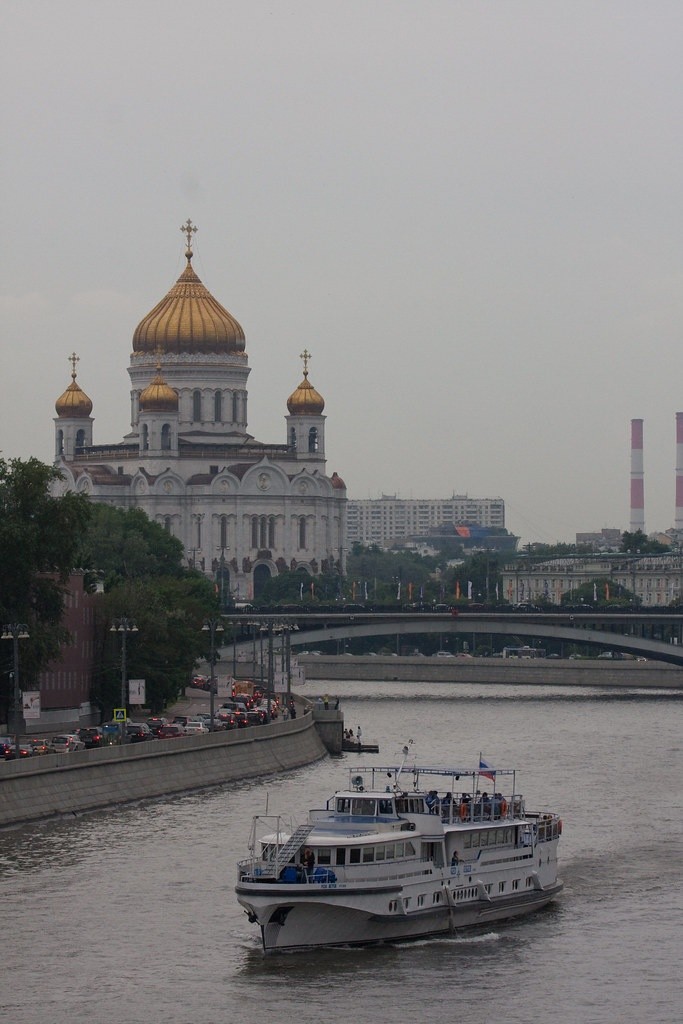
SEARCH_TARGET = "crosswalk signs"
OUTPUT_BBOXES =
[116,709,127,720]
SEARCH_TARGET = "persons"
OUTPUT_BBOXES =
[282,694,339,721]
[425,790,507,822]
[344,725,362,745]
[452,851,467,865]
[303,848,315,874]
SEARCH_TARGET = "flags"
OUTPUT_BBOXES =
[479,757,496,781]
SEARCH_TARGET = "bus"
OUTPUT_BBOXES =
[503,648,546,659]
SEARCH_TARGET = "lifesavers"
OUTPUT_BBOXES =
[558,820,562,834]
[460,804,466,819]
[502,799,507,816]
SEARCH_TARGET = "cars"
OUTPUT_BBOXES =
[238,649,647,657]
[235,604,630,613]
[1,674,280,758]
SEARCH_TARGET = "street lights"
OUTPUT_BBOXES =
[110,619,138,746]
[0,622,30,761]
[627,547,641,612]
[248,618,300,722]
[217,545,231,599]
[201,618,224,732]
[336,548,349,600]
[228,619,241,680]
[188,547,202,567]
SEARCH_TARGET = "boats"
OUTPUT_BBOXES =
[235,762,563,951]
